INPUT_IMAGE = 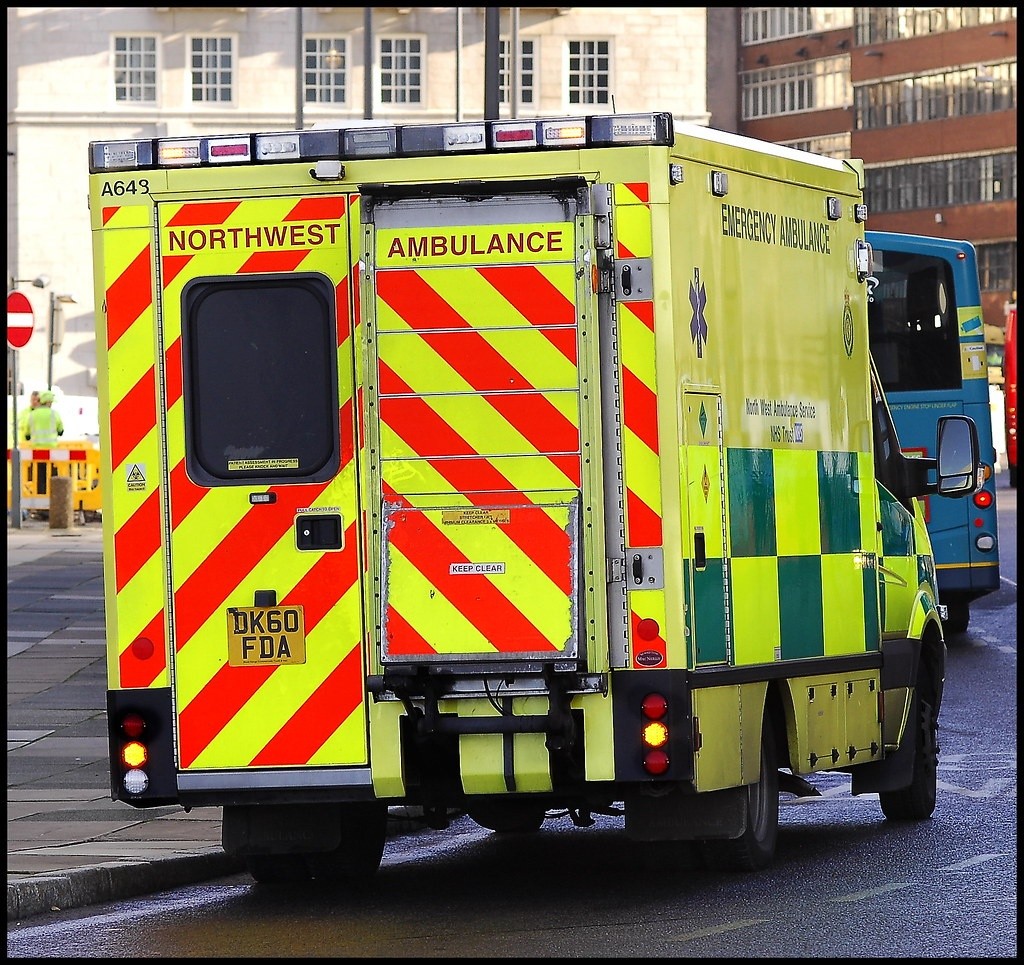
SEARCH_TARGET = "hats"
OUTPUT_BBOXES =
[39,391,54,405]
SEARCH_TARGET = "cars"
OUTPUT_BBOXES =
[1001,304,1019,488]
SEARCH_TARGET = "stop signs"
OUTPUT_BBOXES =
[6,290,35,350]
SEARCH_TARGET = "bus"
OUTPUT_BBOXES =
[862,230,1003,639]
[982,324,1007,390]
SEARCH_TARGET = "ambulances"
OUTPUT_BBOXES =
[87,113,980,898]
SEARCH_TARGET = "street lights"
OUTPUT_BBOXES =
[9,275,51,529]
[46,292,78,393]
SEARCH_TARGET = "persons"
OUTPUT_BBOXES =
[17,390,63,520]
